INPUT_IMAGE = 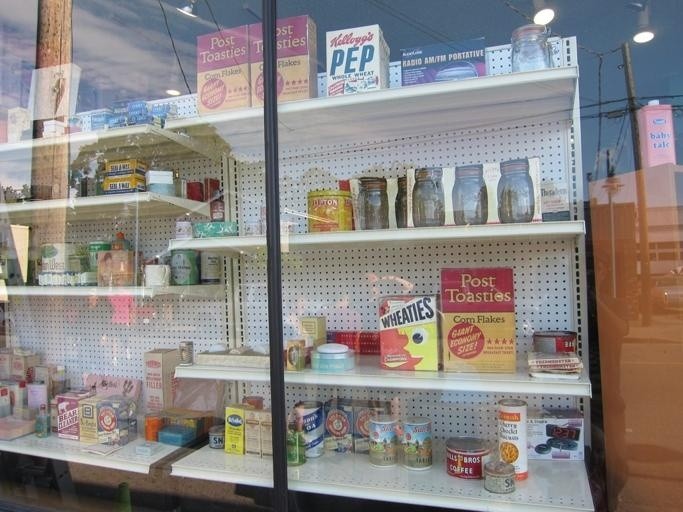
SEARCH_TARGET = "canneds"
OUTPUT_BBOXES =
[305,189,352,231]
[399,416,434,468]
[532,329,577,354]
[294,400,325,458]
[445,434,496,480]
[498,398,529,480]
[367,415,397,467]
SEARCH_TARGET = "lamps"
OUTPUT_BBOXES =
[176,0,199,18]
[531,0,555,26]
[164,53,182,96]
[632,4,654,45]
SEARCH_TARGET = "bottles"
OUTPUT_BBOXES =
[111,231,131,251]
[356,158,535,231]
[510,23,554,74]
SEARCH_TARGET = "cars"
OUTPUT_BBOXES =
[653,274,683,312]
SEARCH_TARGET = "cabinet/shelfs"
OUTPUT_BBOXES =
[587,164,683,510]
[161,66,597,512]
[0,122,216,477]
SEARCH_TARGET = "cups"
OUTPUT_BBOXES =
[178,341,194,365]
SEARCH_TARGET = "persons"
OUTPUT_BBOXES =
[536,256,630,512]
